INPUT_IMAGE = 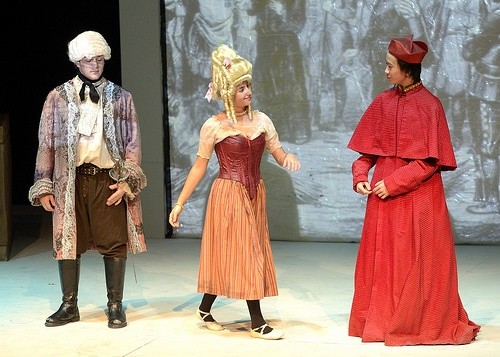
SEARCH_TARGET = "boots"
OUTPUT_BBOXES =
[103,256,127,328]
[44,257,80,327]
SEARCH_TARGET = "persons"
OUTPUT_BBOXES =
[348,32,481,346]
[165,0,500,213]
[169,43,301,341]
[28,31,148,328]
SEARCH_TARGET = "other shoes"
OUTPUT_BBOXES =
[195,306,225,331]
[249,323,283,340]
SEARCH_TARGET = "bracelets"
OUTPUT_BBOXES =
[174,203,183,209]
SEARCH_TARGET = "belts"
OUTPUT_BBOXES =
[76,166,110,176]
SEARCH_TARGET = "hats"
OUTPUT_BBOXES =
[388,34,429,64]
